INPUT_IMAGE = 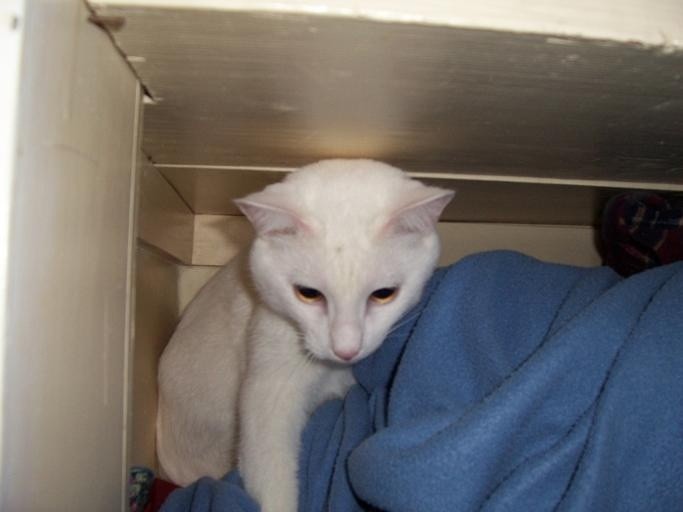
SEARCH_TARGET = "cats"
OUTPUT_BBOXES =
[154,158,457,512]
